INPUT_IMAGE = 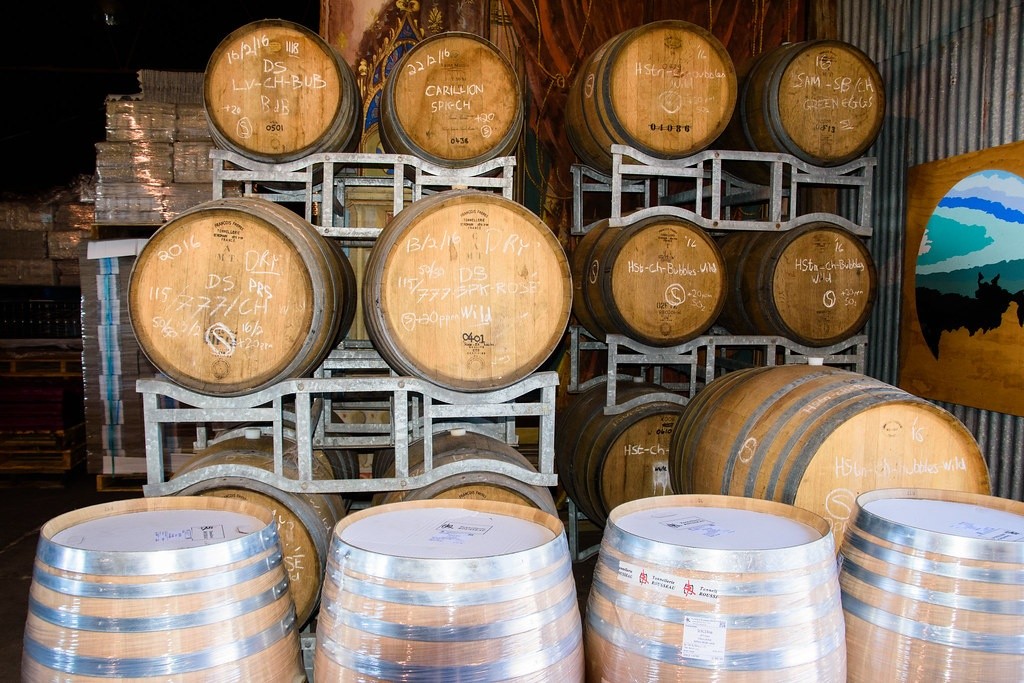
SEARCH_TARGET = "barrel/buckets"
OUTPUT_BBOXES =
[169,402,360,632]
[563,19,738,180]
[667,364,993,551]
[371,417,559,519]
[126,196,359,399]
[313,497,585,683]
[554,380,685,530]
[724,37,887,188]
[20,495,310,683]
[360,189,574,395]
[583,493,848,683]
[835,487,1024,683]
[572,210,731,349]
[378,30,524,192]
[201,18,365,192]
[715,220,878,356]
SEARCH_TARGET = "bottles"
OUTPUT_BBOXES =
[0,299,81,338]
